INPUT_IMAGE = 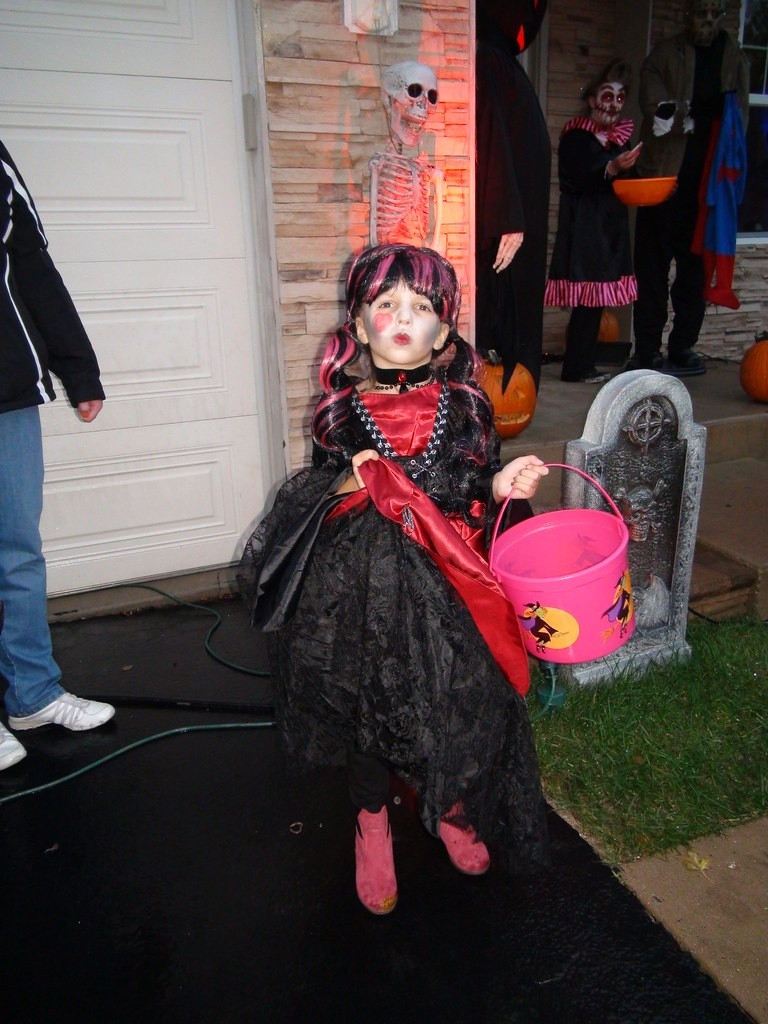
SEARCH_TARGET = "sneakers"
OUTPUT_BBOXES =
[9,692,115,731]
[0,721,27,770]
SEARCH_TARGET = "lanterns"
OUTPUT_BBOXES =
[473,359,536,439]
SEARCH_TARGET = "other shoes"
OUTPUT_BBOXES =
[624,355,672,375]
[580,374,605,382]
[439,800,490,875]
[354,804,398,915]
[595,371,611,381]
[668,348,707,375]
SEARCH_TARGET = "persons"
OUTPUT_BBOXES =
[0,138,115,772]
[224,241,549,915]
[541,57,644,385]
[625,0,751,378]
[475,37,526,366]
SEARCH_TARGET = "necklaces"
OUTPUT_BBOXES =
[372,359,435,391]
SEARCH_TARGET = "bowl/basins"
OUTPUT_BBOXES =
[613,177,678,206]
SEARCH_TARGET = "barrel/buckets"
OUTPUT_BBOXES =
[488,461,636,663]
